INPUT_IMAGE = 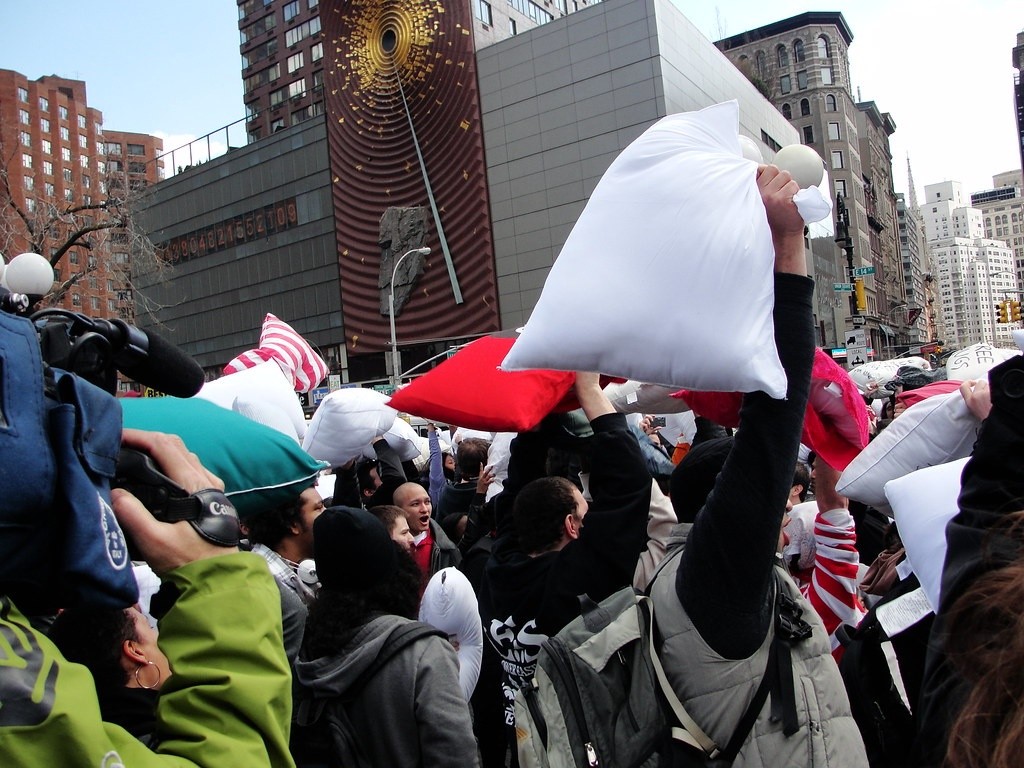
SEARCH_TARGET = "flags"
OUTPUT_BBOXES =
[908,307,922,326]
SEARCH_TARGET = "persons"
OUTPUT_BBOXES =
[292,506,485,768]
[0,166,1024,768]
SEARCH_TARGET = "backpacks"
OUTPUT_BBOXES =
[511,541,813,768]
[833,579,921,711]
[0,312,146,618]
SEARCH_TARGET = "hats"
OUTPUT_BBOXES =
[314,504,397,607]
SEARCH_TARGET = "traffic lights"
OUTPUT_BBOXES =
[1010,299,1022,322]
[996,302,1008,324]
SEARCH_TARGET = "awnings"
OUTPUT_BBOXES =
[823,347,874,363]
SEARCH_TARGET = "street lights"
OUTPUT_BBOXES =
[389,245,432,392]
